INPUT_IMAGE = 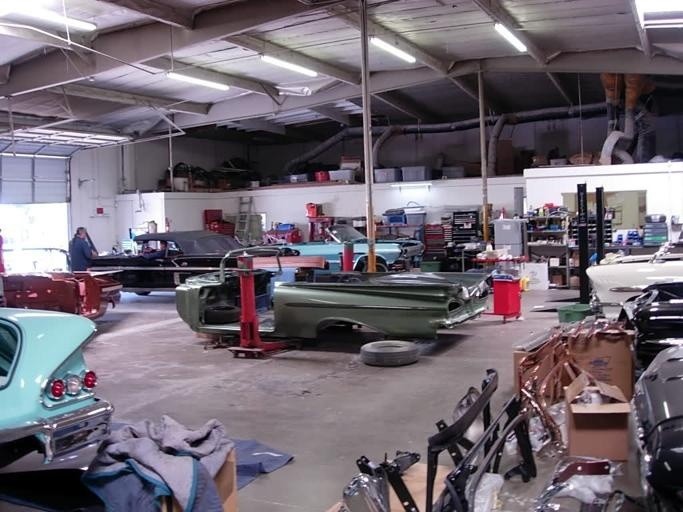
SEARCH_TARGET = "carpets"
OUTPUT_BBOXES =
[79,413,294,511]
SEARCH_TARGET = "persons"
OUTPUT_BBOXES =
[67,227,96,273]
[157,240,169,250]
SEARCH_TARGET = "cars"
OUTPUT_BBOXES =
[1,307,115,472]
[174,248,494,343]
[0,268,124,321]
[616,279,682,374]
[268,222,428,274]
[586,252,683,323]
[90,229,300,296]
[629,346,683,492]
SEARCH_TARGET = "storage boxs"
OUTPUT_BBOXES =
[403,211,426,226]
[290,155,464,188]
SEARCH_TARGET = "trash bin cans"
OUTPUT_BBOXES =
[557,304,591,323]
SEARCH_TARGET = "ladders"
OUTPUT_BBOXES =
[234,196,252,242]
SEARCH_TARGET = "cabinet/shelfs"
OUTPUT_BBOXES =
[522,215,569,288]
